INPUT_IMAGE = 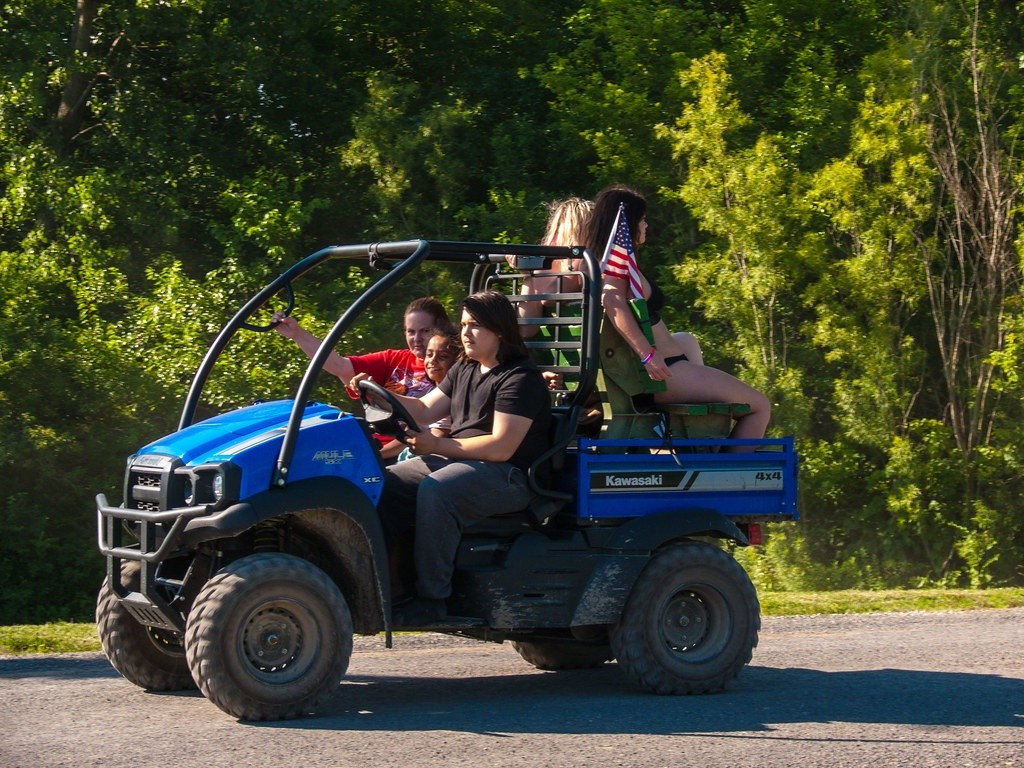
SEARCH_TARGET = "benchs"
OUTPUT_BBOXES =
[524,298,751,453]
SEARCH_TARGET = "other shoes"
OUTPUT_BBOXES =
[391,588,414,606]
[392,595,455,627]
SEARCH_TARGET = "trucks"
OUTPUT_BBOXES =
[95,240,796,723]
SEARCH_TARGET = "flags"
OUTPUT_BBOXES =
[605,208,646,299]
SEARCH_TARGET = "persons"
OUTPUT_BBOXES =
[270,298,450,464]
[380,330,559,462]
[541,190,779,453]
[349,295,553,627]
[505,197,596,337]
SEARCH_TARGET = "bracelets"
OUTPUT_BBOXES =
[641,347,655,365]
